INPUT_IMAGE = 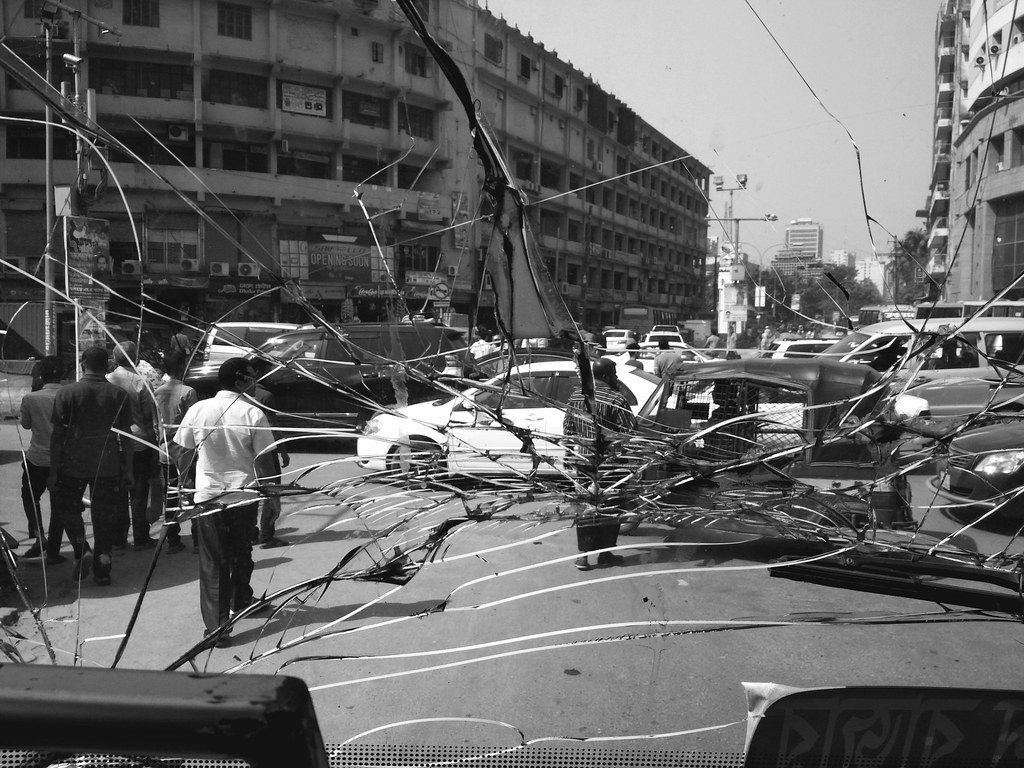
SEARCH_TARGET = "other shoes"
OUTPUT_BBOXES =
[135,538,159,551]
[166,542,185,554]
[203,630,230,647]
[72,551,93,581]
[112,541,132,550]
[598,552,624,566]
[261,538,289,548]
[246,598,271,616]
[46,555,73,565]
[574,556,588,569]
[251,537,258,545]
[25,537,48,557]
[193,545,199,553]
[93,571,111,585]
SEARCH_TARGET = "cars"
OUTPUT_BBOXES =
[352,359,721,492]
[882,374,1024,463]
[939,421,1024,525]
[469,322,845,383]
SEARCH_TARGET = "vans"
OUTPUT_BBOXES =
[807,316,1024,391]
[202,321,318,366]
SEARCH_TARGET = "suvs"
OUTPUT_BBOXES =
[187,320,480,446]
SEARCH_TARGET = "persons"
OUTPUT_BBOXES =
[469,324,498,360]
[172,357,280,648]
[153,354,198,555]
[46,347,135,586]
[625,343,643,371]
[18,356,89,566]
[653,338,684,410]
[665,379,742,481]
[251,357,289,548]
[170,326,191,368]
[705,319,826,360]
[104,339,159,552]
[561,356,638,571]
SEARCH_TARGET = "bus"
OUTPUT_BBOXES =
[915,297,1024,359]
[858,304,920,326]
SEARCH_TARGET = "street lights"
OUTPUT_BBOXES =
[701,213,779,265]
[712,173,748,244]
